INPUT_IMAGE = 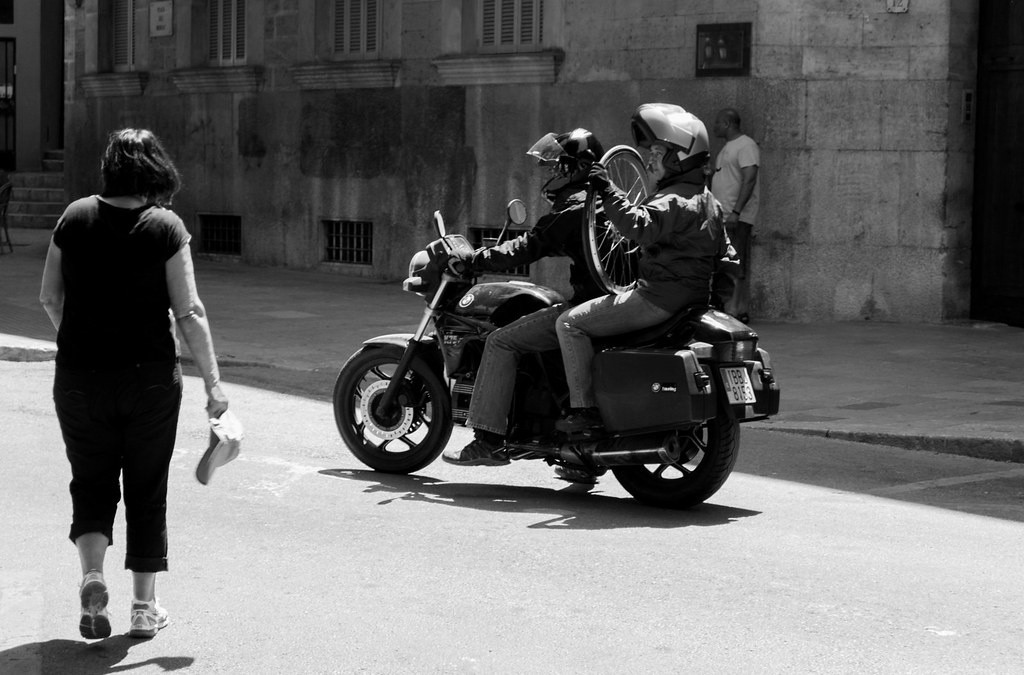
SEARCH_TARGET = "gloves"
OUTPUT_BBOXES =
[588,160,613,199]
[447,250,473,278]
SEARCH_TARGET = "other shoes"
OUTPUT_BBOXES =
[731,312,750,324]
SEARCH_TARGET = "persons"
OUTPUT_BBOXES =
[39,127,229,639]
[441,103,760,483]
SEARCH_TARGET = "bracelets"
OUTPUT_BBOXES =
[732,210,741,216]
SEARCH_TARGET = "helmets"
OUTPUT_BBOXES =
[632,102,710,176]
[537,127,605,210]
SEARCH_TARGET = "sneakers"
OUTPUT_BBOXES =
[79,570,112,640]
[442,439,511,466]
[555,407,600,431]
[131,597,169,637]
[555,464,597,483]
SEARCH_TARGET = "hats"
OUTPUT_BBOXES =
[195,409,243,485]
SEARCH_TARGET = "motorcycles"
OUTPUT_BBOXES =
[330,197,781,512]
